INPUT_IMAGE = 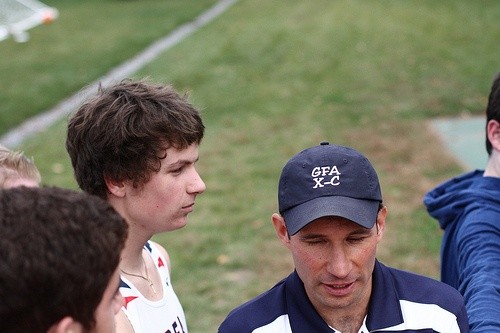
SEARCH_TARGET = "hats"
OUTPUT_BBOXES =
[278,143,382,236]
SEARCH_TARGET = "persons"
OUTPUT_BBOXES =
[0,145,43,192]
[422,72,500,333]
[0,185,127,333]
[216,141,470,333]
[65,79,207,333]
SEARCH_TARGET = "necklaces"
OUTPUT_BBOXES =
[118,254,158,297]
